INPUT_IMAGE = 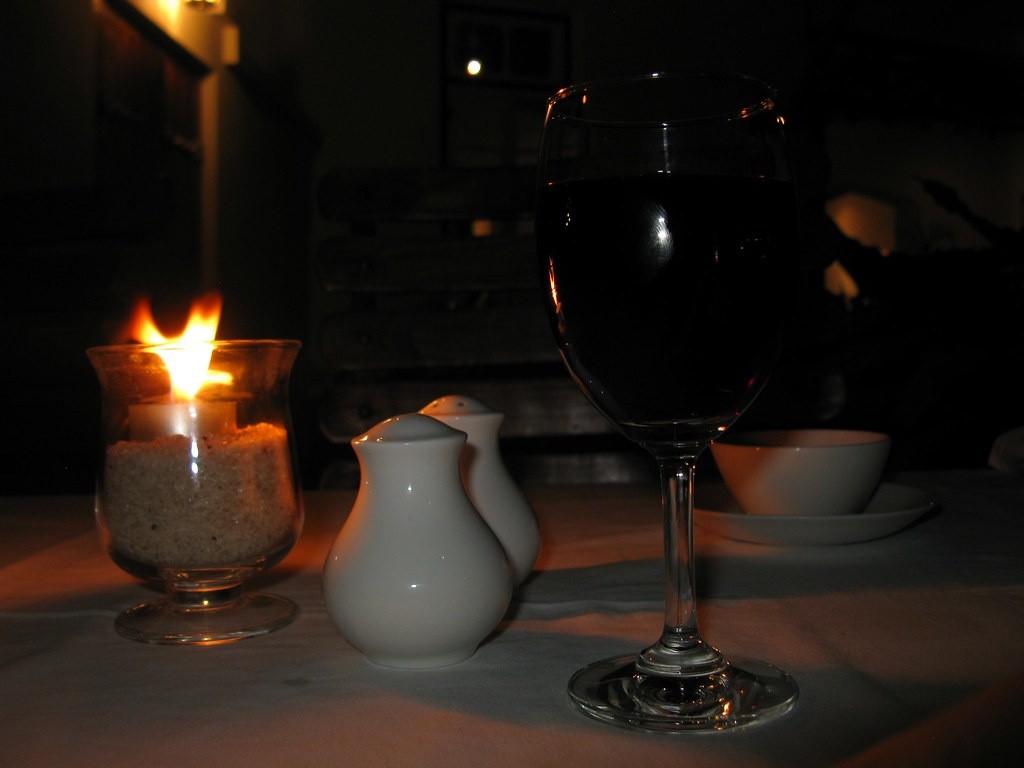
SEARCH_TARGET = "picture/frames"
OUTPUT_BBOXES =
[439,2,572,92]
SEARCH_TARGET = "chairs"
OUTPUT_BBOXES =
[314,163,676,491]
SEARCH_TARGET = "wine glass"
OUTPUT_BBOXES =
[537,66,810,738]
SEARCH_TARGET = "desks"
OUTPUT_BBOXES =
[0,485,1024,768]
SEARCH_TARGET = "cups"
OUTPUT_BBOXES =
[83,337,306,648]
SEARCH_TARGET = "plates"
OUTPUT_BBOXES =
[693,484,937,546]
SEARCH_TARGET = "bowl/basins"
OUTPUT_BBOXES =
[705,428,888,516]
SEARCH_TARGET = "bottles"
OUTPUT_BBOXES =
[320,413,514,666]
[417,394,542,586]
